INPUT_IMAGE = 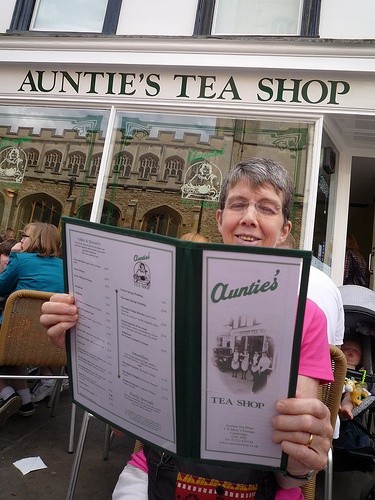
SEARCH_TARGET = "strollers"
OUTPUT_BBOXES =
[331,284,375,474]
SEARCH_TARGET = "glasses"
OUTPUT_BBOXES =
[21,234,30,238]
[223,199,283,217]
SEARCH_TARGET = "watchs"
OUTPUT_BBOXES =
[281,466,317,482]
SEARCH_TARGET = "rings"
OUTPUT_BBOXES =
[305,434,313,447]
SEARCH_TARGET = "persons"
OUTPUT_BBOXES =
[344,234,367,288]
[0,240,20,273]
[31,366,70,403]
[337,340,371,420]
[39,157,335,500]
[273,232,297,250]
[0,222,66,421]
[0,228,16,243]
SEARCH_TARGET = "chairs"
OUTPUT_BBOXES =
[135,343,348,500]
[65,409,111,500]
[0,290,77,453]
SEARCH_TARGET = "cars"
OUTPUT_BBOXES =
[213,347,234,371]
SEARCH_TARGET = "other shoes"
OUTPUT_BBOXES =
[16,402,36,417]
[0,393,23,424]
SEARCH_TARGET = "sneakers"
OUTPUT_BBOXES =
[30,380,63,402]
[62,378,69,391]
